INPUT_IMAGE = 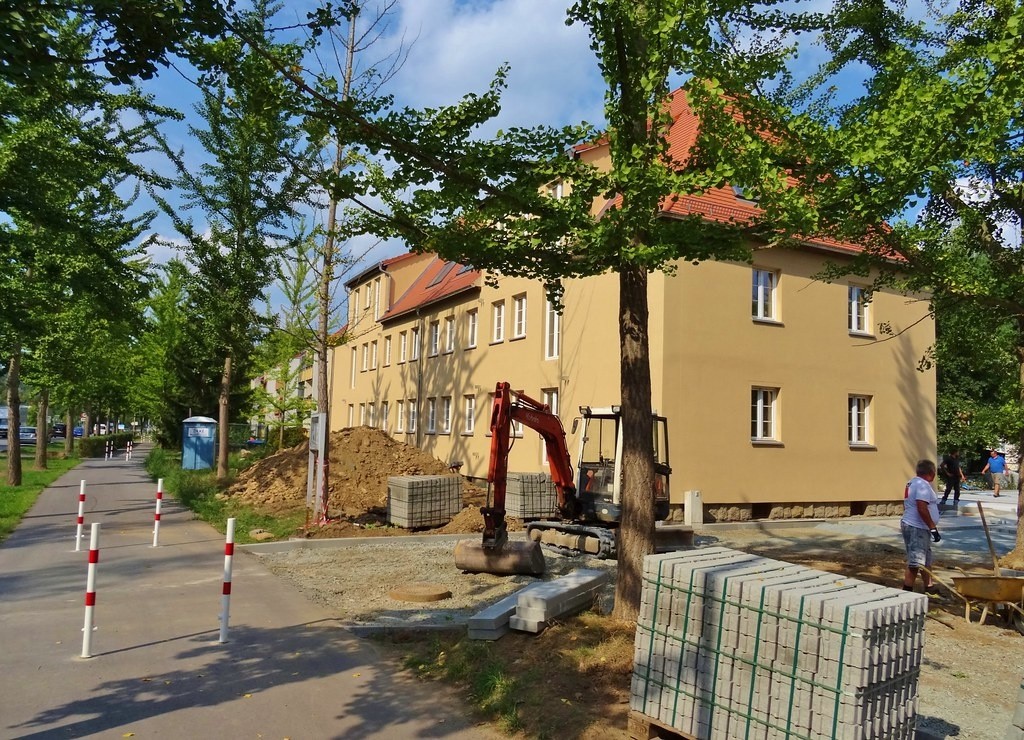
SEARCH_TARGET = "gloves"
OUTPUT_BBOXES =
[931,528,941,542]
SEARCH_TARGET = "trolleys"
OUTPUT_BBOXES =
[909,562,1024,636]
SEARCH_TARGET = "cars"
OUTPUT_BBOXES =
[93,424,112,437]
[19,427,37,444]
[0,418,9,436]
[73,427,83,437]
[52,423,66,438]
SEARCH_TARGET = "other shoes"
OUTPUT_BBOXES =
[993,493,999,497]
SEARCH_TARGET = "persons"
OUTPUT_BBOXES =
[981,450,1009,497]
[900,460,942,598]
[940,446,964,511]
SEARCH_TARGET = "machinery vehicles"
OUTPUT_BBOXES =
[451,379,695,577]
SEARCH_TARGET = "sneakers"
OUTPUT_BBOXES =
[925,591,951,604]
